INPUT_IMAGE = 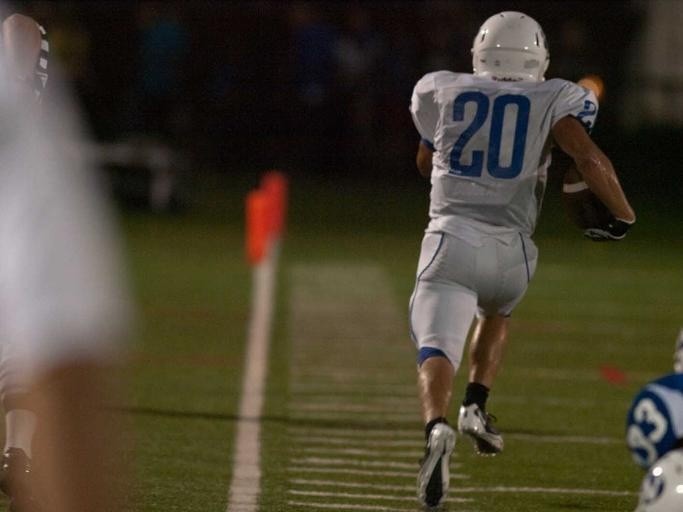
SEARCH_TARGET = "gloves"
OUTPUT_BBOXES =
[583,215,637,243]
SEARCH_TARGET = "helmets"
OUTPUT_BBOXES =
[470,10,551,83]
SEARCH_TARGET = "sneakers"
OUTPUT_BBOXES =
[458,402,504,457]
[415,421,459,511]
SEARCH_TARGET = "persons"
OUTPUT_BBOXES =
[622,327,683,512]
[407,11,636,508]
[0,6,132,512]
